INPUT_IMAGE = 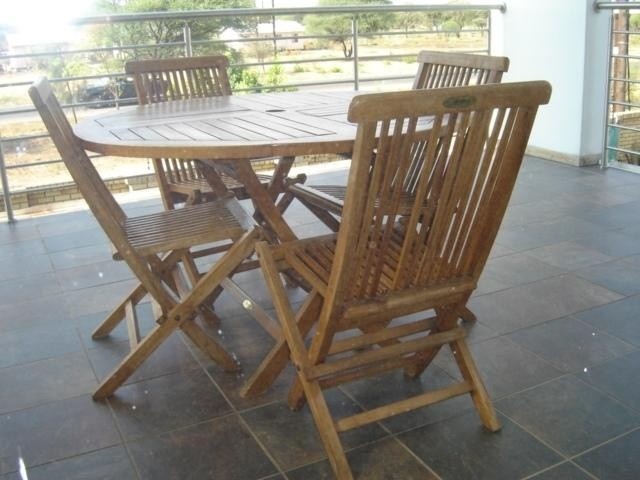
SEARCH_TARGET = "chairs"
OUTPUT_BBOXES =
[125,57,316,322]
[238,81,552,480]
[28,79,253,405]
[289,52,514,352]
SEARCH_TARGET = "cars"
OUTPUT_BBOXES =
[0,25,74,74]
[79,74,172,109]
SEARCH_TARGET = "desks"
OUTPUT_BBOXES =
[68,90,455,409]
